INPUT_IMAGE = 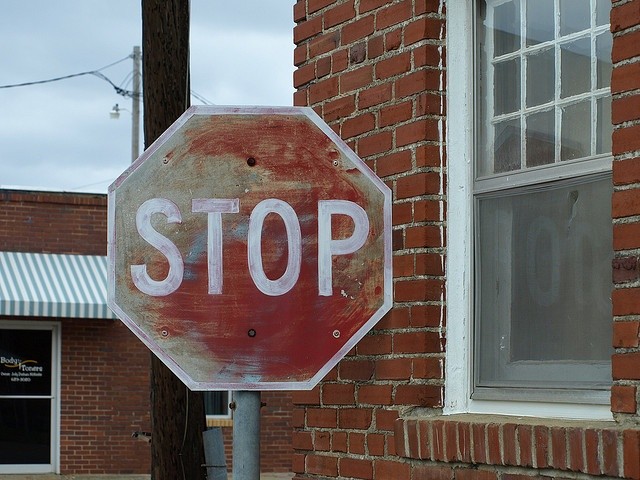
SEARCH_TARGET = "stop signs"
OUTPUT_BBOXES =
[108,105,393,391]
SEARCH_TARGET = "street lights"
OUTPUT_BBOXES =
[109,103,140,163]
